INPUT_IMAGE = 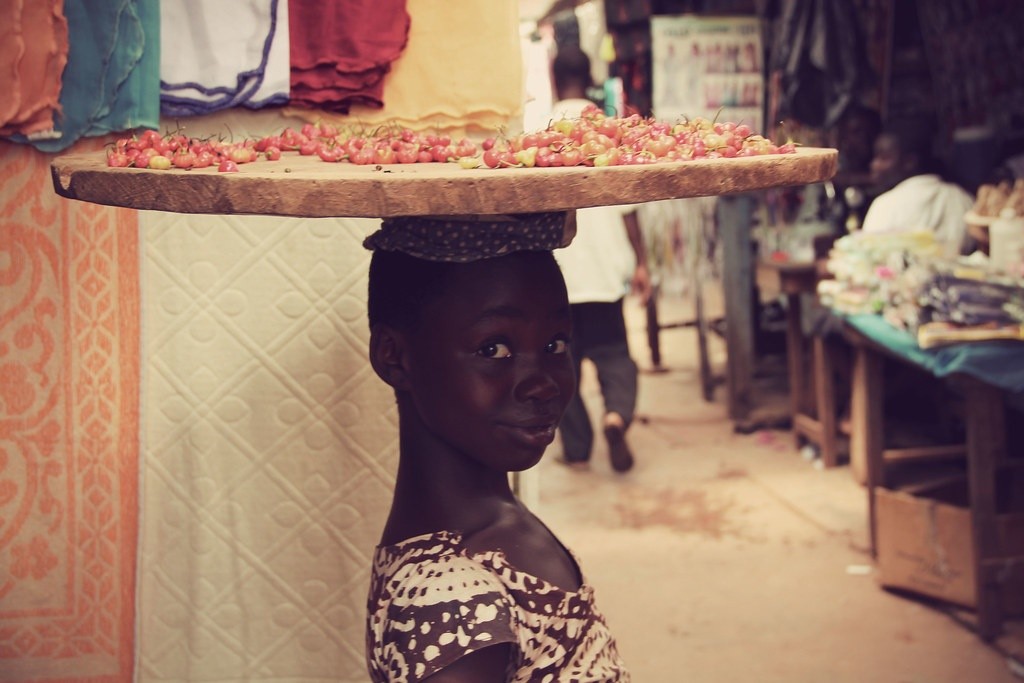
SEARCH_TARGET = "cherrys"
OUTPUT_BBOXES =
[104,101,802,173]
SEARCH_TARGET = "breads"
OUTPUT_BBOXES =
[967,178,1024,244]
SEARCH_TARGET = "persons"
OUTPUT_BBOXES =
[366,251,637,683]
[524,43,655,474]
[707,108,975,451]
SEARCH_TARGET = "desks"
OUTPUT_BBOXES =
[754,252,1024,515]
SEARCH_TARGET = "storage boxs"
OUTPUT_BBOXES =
[874,459,1024,617]
